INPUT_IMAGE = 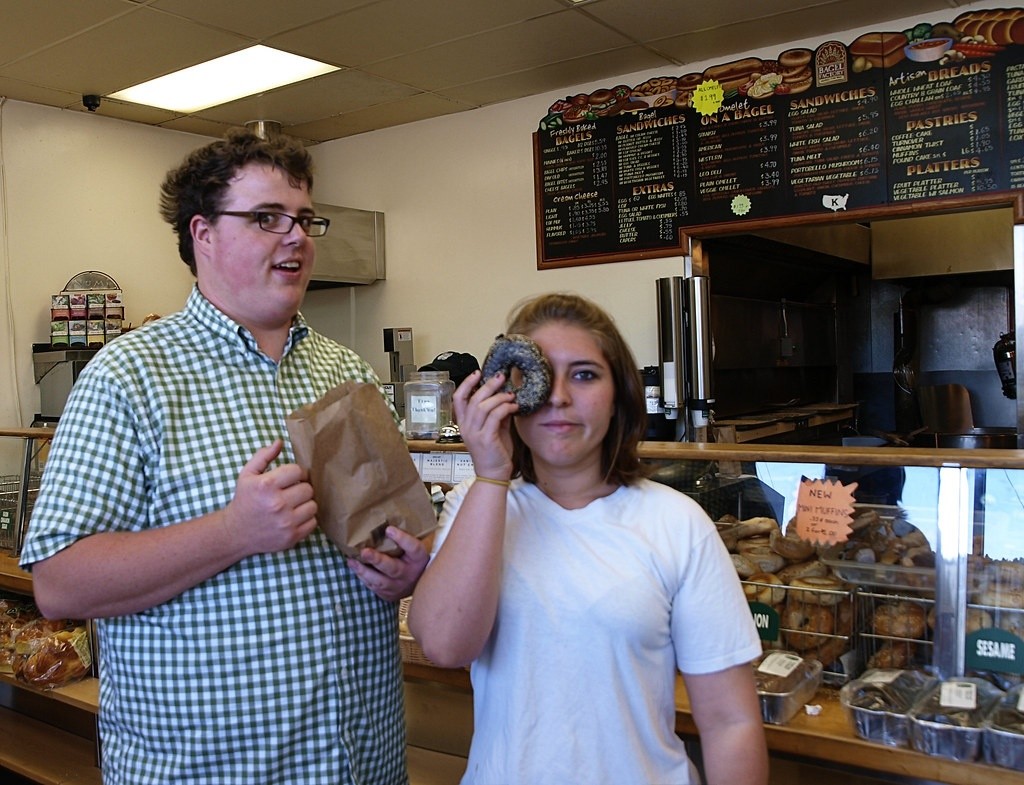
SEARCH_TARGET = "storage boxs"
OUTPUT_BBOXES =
[52,295,124,350]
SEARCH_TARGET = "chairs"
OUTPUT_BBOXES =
[916,383,975,428]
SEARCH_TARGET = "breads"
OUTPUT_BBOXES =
[873,554,1024,688]
[0,598,91,688]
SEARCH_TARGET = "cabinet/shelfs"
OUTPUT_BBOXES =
[0,548,1024,785]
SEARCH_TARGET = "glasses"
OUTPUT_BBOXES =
[204,212,330,237]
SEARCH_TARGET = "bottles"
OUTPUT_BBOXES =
[402,370,456,442]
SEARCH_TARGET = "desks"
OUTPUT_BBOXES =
[921,428,1016,449]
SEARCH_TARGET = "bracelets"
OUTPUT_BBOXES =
[475,476,511,486]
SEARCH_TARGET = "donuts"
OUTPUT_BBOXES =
[480,333,553,416]
[709,515,854,668]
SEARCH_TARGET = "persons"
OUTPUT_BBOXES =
[407,292,768,785]
[21,131,436,785]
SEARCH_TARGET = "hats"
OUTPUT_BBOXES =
[417,351,482,390]
